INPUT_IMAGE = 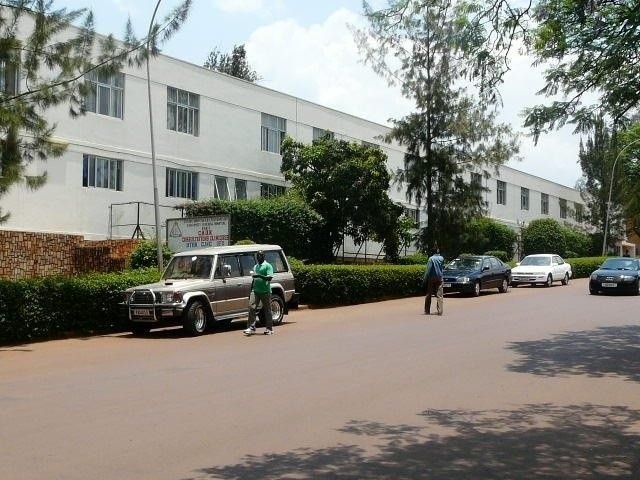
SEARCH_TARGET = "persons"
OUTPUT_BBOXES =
[623,249,630,257]
[423,246,445,316]
[243,250,274,335]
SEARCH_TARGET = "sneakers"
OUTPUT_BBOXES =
[244,328,255,334]
[264,329,274,335]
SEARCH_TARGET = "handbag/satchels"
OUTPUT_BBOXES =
[429,273,441,290]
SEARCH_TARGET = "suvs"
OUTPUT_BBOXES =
[119,244,296,335]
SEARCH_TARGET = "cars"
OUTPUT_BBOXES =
[442,255,512,296]
[510,254,572,287]
[588,257,639,294]
